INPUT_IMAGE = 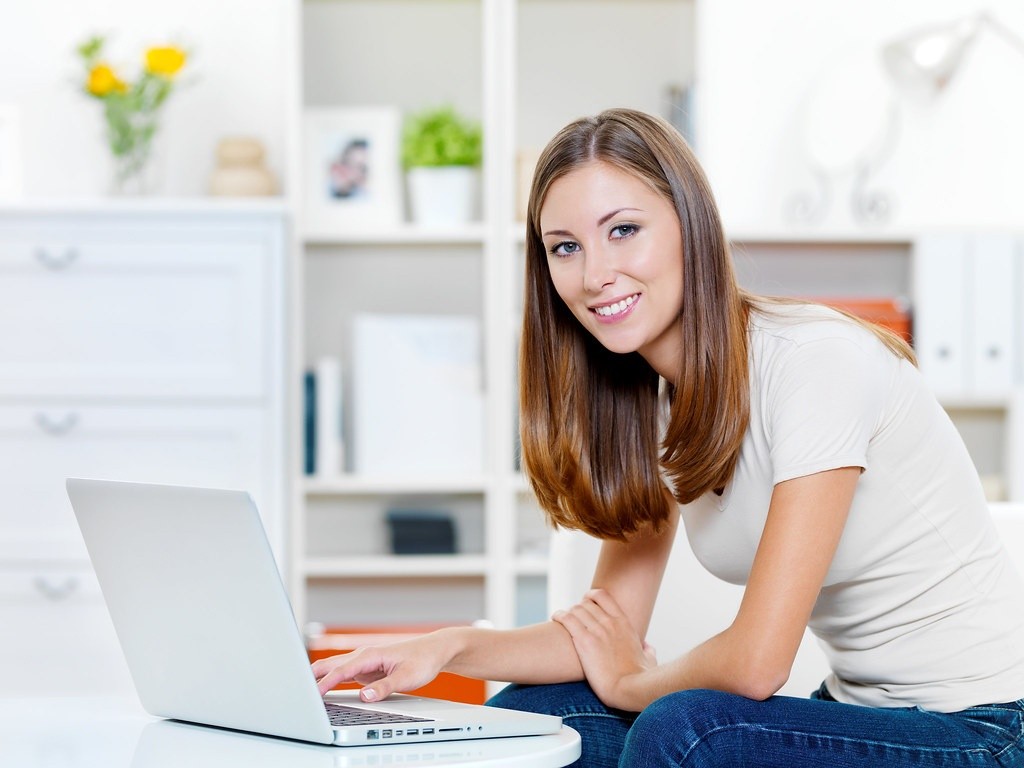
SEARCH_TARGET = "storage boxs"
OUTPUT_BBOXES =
[305,620,489,706]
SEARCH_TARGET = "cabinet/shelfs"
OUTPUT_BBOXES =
[292,0,1022,705]
[0,202,295,707]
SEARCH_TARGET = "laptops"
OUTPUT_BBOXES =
[63,477,563,746]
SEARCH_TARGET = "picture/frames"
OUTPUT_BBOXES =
[306,105,411,231]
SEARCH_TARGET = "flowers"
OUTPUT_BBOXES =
[85,36,183,175]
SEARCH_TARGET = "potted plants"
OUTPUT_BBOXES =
[399,105,484,223]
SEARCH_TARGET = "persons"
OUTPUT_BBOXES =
[311,108,1024,768]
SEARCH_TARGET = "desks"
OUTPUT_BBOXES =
[69,709,584,767]
